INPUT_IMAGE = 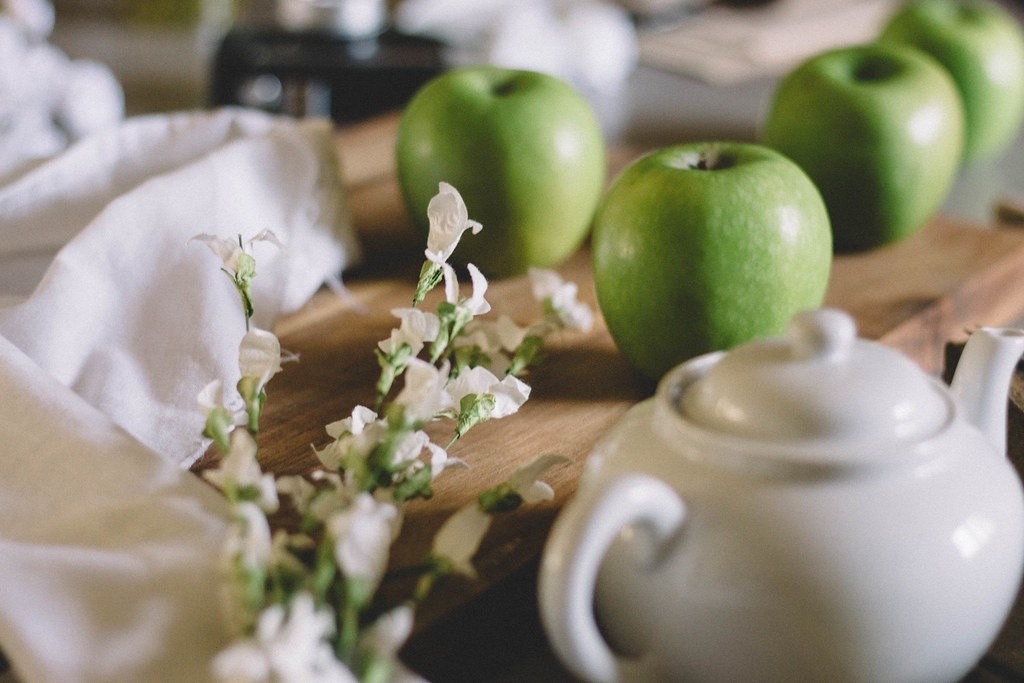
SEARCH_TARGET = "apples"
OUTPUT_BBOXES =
[394,0,1024,383]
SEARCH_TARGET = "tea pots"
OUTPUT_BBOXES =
[537,309,1024,682]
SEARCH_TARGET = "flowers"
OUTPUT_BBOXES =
[188,179,592,681]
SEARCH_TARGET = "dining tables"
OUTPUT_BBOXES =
[0,95,1023,681]
